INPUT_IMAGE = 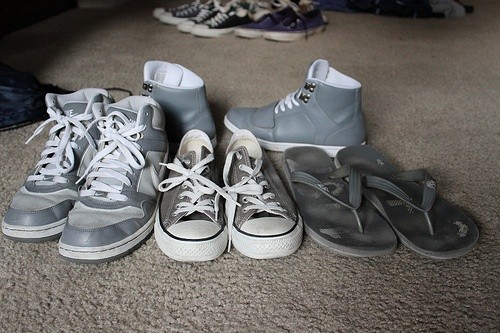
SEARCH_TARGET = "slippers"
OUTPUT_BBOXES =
[281,146,398,257]
[334,145,480,259]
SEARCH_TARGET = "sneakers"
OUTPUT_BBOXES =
[154,129,242,262]
[139,60,218,148]
[264,4,328,43]
[159,0,204,25]
[58,95,170,264]
[214,129,303,260]
[178,0,225,33]
[224,59,367,158]
[154,2,190,20]
[1,87,115,243]
[191,0,252,37]
[234,6,297,39]
